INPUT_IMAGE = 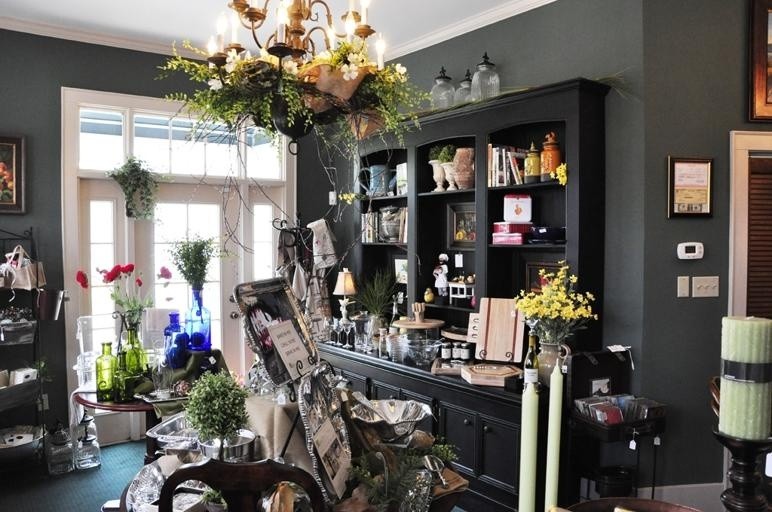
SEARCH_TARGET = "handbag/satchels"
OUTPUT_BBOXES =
[5,245,47,291]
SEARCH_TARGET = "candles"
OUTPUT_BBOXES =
[716,316,772,438]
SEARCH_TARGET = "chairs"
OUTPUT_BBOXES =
[158,458,330,512]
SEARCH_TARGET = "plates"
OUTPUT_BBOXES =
[527,240,567,245]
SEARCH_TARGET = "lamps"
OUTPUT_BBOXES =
[159,2,391,159]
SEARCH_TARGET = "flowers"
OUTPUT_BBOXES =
[75,260,172,366]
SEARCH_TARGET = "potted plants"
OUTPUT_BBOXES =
[181,371,252,512]
[167,232,235,350]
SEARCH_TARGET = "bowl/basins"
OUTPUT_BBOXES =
[529,227,567,239]
[386,333,410,361]
[409,339,440,365]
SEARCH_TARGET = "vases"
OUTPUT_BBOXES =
[125,322,147,374]
[96,342,118,401]
[114,350,133,402]
[164,312,186,368]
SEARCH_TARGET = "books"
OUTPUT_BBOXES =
[573,393,668,426]
[486,144,528,187]
[361,207,408,244]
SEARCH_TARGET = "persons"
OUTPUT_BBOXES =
[432,254,449,298]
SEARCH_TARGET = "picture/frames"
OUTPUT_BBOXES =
[747,1,772,126]
[0,132,28,215]
[665,153,714,218]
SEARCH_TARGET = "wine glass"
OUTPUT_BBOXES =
[323,316,353,348]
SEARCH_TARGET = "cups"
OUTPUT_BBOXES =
[354,334,366,349]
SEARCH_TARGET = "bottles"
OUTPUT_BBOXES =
[524,334,538,392]
[429,49,500,112]
[377,327,408,356]
[442,342,471,363]
[163,312,186,356]
[96,327,146,405]
[48,411,102,476]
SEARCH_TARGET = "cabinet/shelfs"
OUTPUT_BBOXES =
[0,224,47,478]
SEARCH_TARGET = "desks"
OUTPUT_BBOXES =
[239,384,315,479]
[74,382,196,511]
